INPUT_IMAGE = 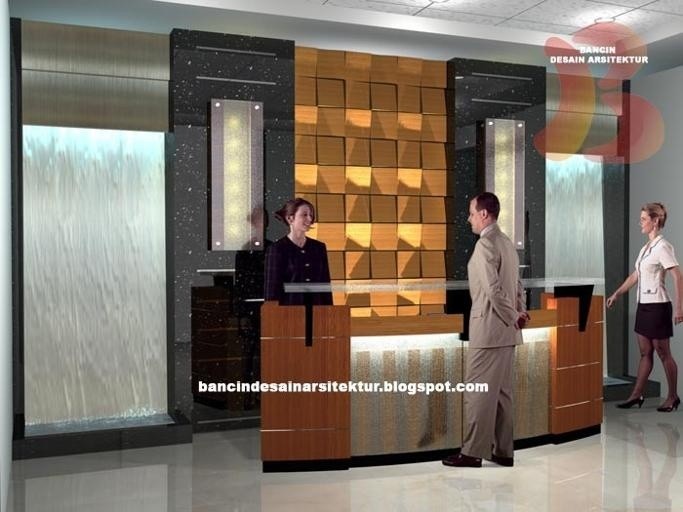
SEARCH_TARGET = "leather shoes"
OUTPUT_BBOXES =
[485,453,513,466]
[442,454,482,467]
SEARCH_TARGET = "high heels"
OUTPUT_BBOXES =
[657,397,680,412]
[615,395,644,408]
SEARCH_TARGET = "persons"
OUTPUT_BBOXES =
[263,198,334,345]
[606,203,683,412]
[442,192,531,467]
